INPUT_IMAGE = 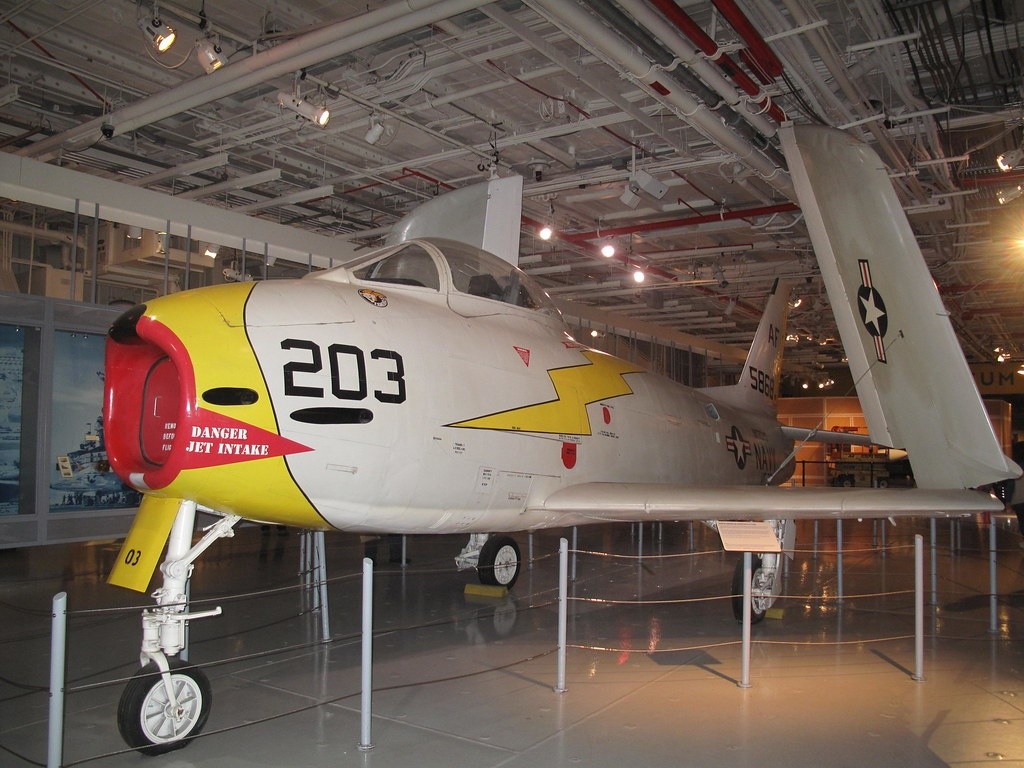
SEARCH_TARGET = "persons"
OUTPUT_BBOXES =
[1006,464,1024,547]
[361,534,412,568]
[260,525,291,536]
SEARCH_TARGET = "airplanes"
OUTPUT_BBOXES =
[98,114,1024,757]
